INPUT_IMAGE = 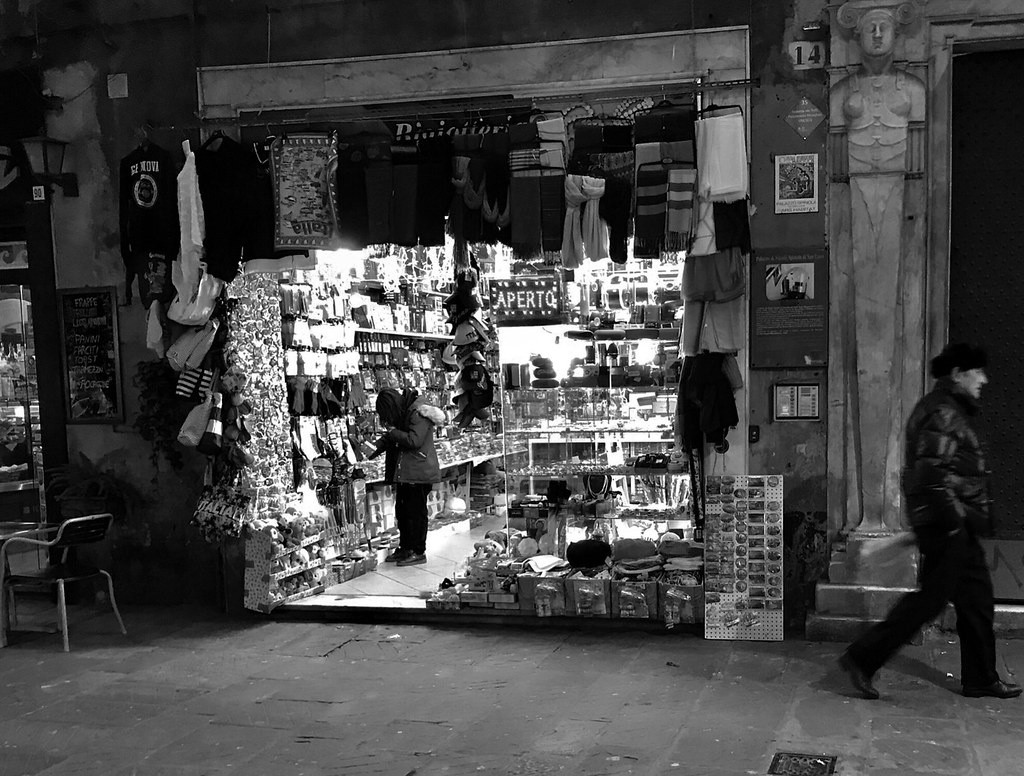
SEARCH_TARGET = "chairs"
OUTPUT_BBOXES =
[0,512,128,652]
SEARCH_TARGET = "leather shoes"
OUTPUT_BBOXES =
[963,679,1024,699]
[836,651,879,700]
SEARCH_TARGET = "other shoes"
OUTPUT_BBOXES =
[397,551,427,566]
[385,547,408,563]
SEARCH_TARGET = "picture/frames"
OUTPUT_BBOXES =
[56,286,126,424]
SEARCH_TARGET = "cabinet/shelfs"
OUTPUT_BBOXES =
[243,501,326,614]
[281,269,704,586]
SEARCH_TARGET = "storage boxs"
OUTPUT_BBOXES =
[425,560,705,624]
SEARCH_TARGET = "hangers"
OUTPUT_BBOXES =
[122,88,746,162]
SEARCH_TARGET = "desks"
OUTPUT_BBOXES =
[0,521,63,634]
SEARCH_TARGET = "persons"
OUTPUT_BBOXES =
[366,386,447,568]
[835,339,1024,702]
[830,8,928,175]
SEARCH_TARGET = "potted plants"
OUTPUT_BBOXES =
[44,450,116,522]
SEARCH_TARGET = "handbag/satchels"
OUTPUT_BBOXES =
[165,270,253,544]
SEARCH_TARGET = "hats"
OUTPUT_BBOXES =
[444,251,494,427]
[931,341,982,377]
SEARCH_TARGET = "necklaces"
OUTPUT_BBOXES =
[586,473,610,499]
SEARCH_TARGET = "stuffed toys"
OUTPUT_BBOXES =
[263,507,329,602]
